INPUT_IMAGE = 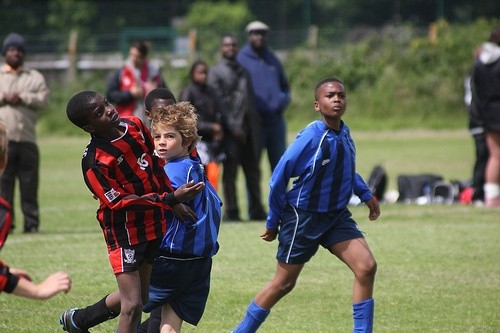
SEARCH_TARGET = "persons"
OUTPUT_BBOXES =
[106,42,168,133]
[0,32,50,232]
[465,30,500,207]
[147,101,223,333]
[144,89,205,177]
[0,123,72,299]
[207,34,270,220]
[238,22,291,218]
[231,78,381,333]
[60,91,205,333]
[179,62,225,191]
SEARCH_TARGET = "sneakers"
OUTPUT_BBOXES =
[59,307,92,333]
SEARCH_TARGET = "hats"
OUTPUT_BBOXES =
[245,20,270,34]
[2,32,27,56]
